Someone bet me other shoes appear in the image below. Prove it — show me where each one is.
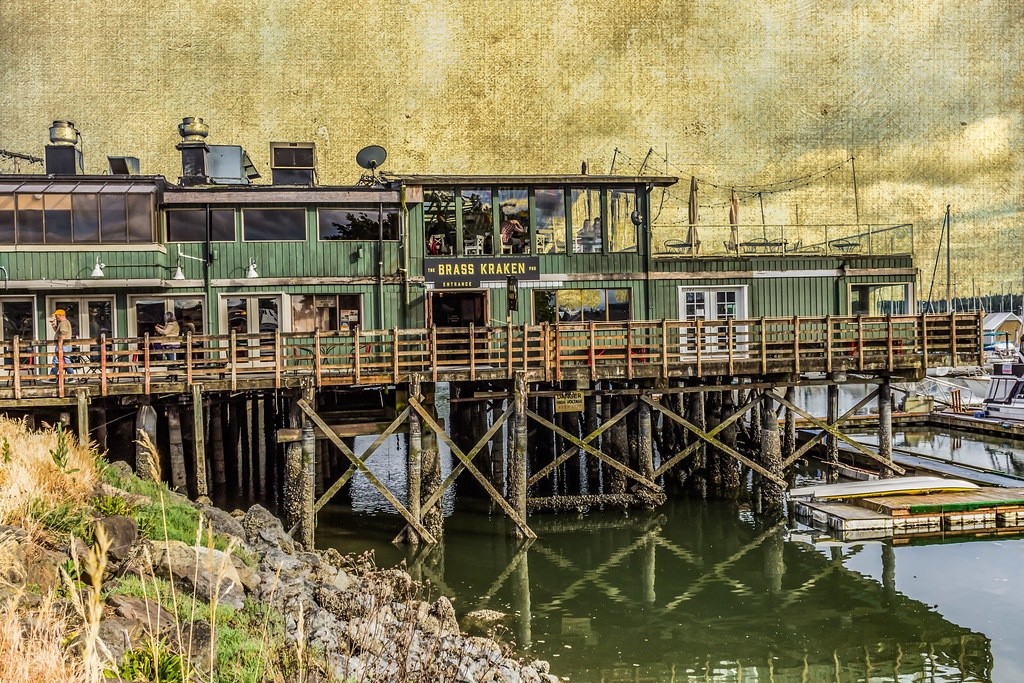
[65,380,75,384]
[43,380,57,384]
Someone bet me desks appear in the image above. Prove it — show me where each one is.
[738,243,788,253]
[667,244,697,254]
[833,243,860,254]
[313,344,342,375]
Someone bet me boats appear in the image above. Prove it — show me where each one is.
[855,331,1024,479]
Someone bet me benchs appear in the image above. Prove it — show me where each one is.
[849,341,904,356]
[587,349,647,367]
[169,352,228,382]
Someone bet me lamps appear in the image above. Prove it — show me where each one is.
[92,255,106,277]
[172,258,186,279]
[245,257,260,278]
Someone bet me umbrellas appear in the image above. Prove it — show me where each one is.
[686,177,698,254]
[730,190,739,251]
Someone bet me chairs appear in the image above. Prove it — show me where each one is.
[292,342,376,374]
[664,236,864,254]
[425,233,603,253]
[6,350,141,382]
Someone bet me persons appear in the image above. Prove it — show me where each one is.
[579,217,601,240]
[183,316,197,359]
[155,311,180,382]
[41,309,76,384]
[501,215,524,253]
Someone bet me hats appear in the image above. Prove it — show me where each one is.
[52,309,66,316]
